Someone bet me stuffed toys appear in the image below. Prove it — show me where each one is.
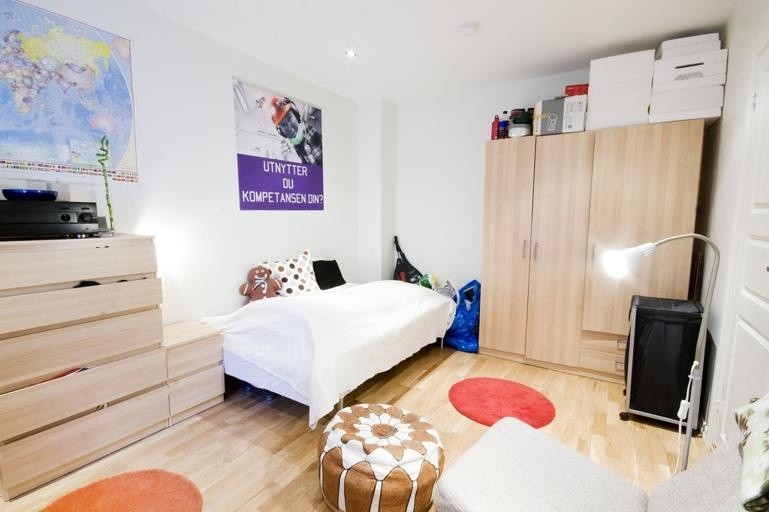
[238,265,284,306]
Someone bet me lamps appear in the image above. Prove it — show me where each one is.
[597,233,722,472]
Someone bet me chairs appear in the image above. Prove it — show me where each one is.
[427,393,769,511]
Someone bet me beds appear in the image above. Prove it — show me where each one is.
[201,278,458,429]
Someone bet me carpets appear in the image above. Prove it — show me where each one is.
[449,377,556,429]
[40,468,204,512]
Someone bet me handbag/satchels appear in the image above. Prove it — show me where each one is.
[443,280,480,353]
[431,279,461,307]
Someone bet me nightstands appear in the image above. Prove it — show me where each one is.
[161,318,226,428]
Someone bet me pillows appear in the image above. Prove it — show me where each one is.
[734,393,769,512]
[312,259,346,290]
[259,250,319,297]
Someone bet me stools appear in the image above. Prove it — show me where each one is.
[317,403,445,512]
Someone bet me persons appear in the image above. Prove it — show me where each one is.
[268,95,323,167]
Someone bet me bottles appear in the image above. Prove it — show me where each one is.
[491,109,509,140]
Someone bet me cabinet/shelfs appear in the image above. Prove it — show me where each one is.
[0,229,167,502]
[477,118,702,385]
[618,295,704,436]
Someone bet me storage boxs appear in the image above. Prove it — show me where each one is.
[532,32,729,136]
